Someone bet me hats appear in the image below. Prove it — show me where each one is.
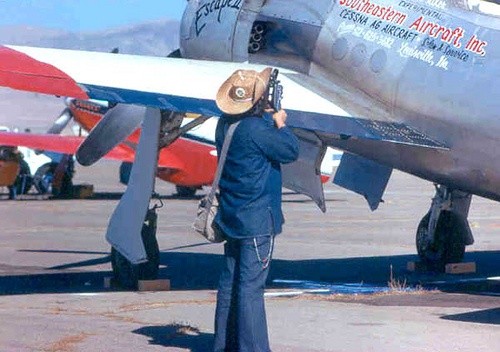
[215,67,273,116]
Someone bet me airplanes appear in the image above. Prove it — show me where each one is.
[0,0,500,287]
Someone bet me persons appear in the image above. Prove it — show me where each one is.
[0,145,34,200]
[209,67,301,352]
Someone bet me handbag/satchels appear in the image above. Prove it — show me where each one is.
[192,190,225,243]
